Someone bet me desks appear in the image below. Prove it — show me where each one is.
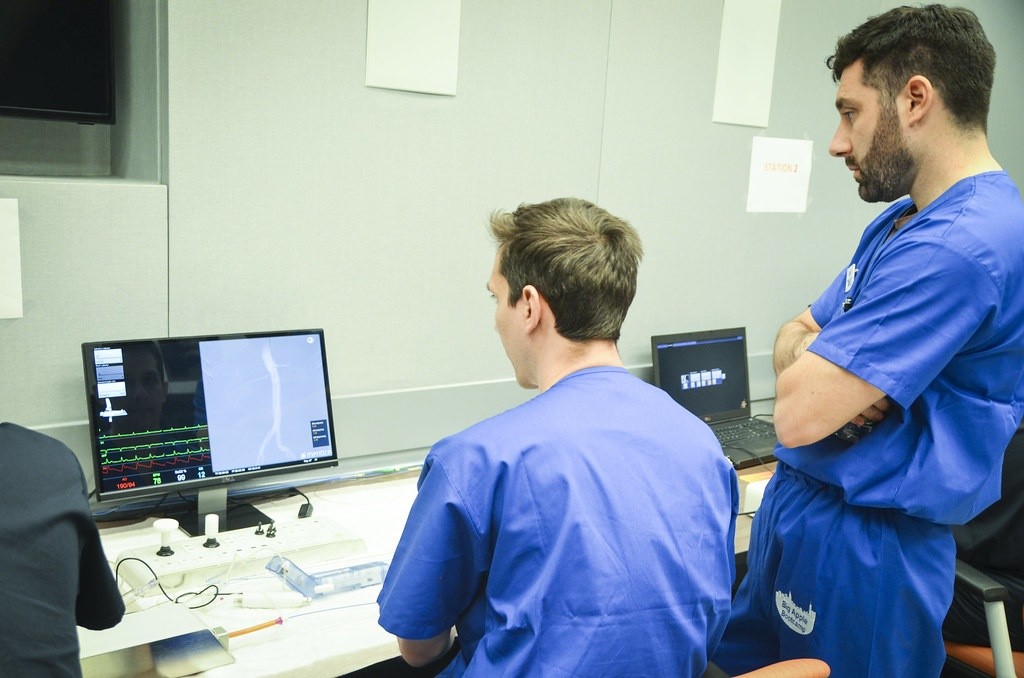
[76,455,779,678]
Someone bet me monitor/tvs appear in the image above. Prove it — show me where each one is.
[82,329,339,539]
[0,0,117,125]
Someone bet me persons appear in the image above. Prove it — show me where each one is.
[720,4,1024,678]
[0,421,126,678]
[376,199,739,678]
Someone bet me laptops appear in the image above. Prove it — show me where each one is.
[652,327,784,470]
